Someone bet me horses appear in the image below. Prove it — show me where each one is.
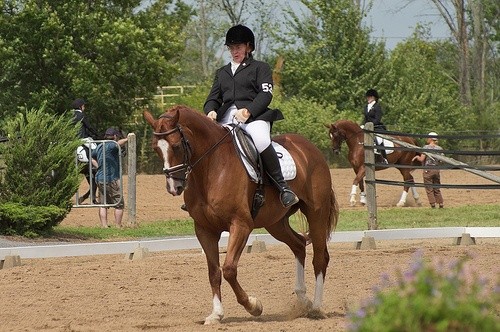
[76,125,126,206]
[139,103,340,325]
[322,117,425,208]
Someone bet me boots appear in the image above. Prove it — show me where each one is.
[259,143,299,209]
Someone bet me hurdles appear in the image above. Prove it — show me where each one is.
[364,121,500,230]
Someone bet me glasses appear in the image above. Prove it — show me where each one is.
[226,45,243,52]
[113,134,119,139]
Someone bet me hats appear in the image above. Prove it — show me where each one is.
[428,132,438,141]
[104,125,123,135]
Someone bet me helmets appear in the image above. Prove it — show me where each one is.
[72,97,87,109]
[365,88,379,103]
[224,24,256,53]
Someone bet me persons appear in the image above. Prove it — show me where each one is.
[417,132,443,208]
[202,24,298,207]
[90,127,128,228]
[360,89,389,165]
[72,98,99,150]
